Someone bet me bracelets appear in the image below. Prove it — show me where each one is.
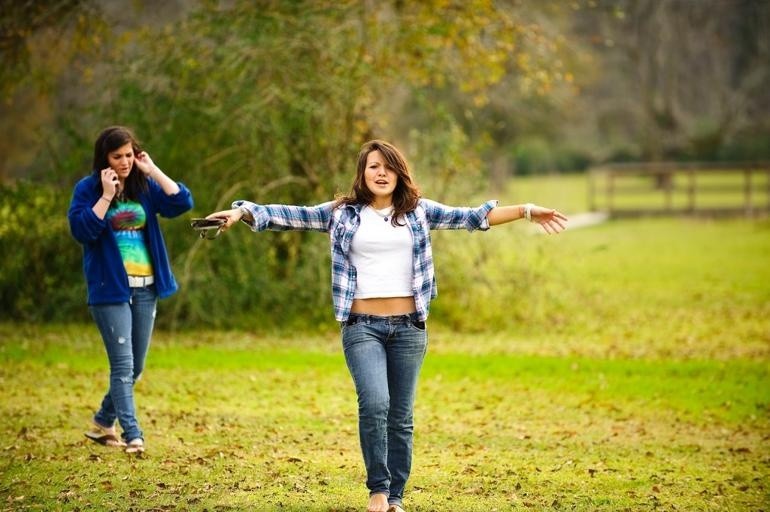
[239,207,248,218]
[526,202,534,221]
[101,196,111,203]
[519,204,526,218]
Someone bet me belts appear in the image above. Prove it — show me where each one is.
[127,275,155,288]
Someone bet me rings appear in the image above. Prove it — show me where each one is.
[111,174,116,178]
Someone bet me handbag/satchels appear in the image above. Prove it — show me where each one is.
[190,217,228,231]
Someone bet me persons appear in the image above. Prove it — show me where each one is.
[68,126,194,452]
[205,140,570,512]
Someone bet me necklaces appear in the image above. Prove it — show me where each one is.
[370,205,394,221]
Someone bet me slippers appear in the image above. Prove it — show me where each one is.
[84,431,126,446]
[126,444,144,454]
